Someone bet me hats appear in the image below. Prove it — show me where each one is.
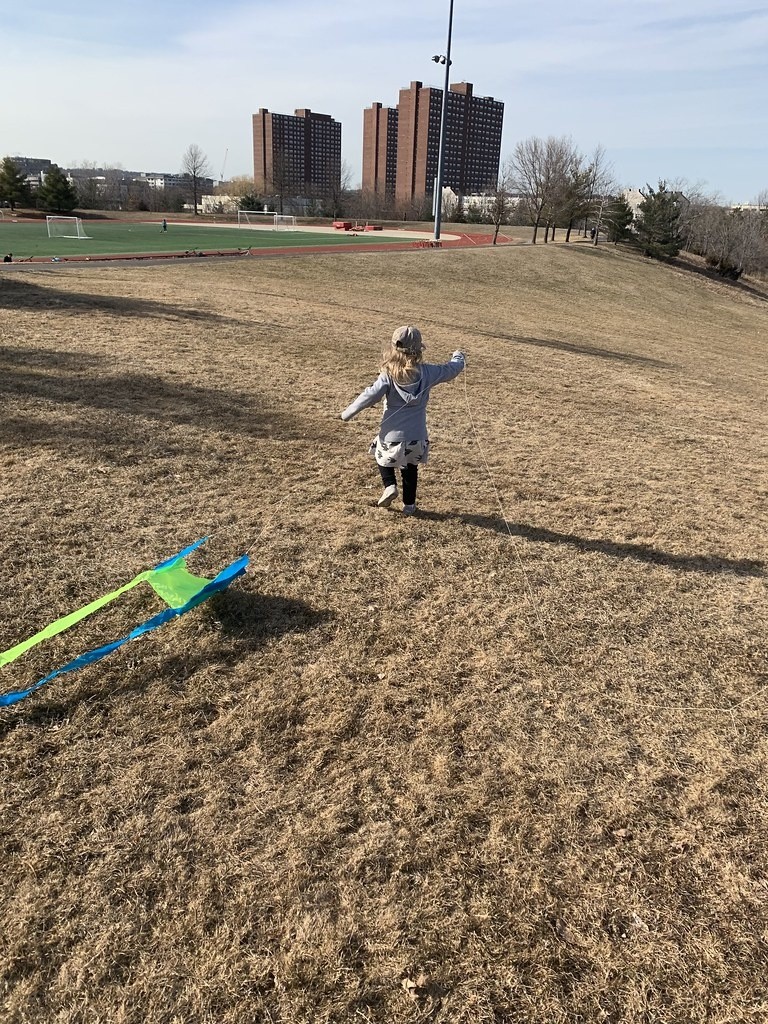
[391,326,422,352]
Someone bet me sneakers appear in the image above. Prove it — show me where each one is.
[403,504,416,515]
[378,485,399,507]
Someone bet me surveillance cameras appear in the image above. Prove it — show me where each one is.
[440,56,445,64]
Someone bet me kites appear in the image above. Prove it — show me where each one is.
[0,533,250,707]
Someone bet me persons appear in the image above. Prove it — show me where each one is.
[162,219,167,233]
[4,253,13,262]
[340,324,466,515]
[589,226,596,244]
